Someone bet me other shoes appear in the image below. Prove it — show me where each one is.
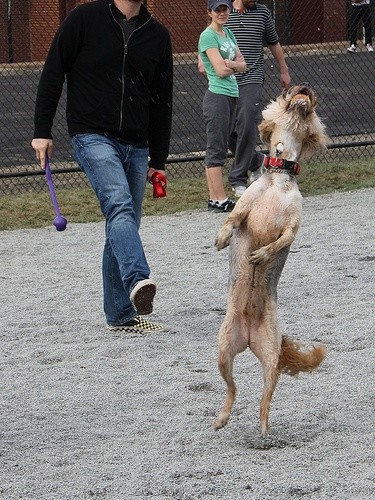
[129,279,156,315]
[366,43,374,52]
[347,44,356,53]
[109,315,163,332]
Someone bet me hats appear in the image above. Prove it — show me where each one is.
[208,0,231,11]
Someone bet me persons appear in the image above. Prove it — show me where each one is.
[198,0,291,197]
[347,0,374,53]
[198,0,246,213]
[31,0,174,332]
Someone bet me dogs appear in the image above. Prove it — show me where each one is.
[210,83,327,439]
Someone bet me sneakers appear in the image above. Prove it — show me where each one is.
[232,185,247,198]
[250,154,266,181]
[208,198,236,213]
